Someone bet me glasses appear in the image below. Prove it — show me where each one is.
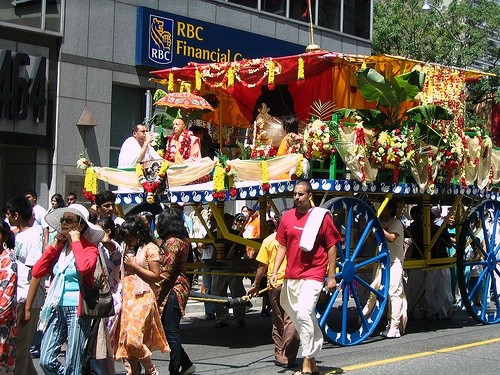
[138,129,149,133]
[60,217,75,224]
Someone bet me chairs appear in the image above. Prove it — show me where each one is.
[310,111,359,180]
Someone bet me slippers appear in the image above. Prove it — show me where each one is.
[291,369,319,375]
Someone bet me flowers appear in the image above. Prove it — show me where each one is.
[76,149,98,202]
[136,58,500,203]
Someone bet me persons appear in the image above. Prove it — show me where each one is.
[191,201,278,325]
[355,203,500,337]
[245,179,343,375]
[0,190,195,375]
[118,118,215,190]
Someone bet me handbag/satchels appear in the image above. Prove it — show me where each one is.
[81,255,115,318]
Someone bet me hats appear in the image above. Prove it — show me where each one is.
[45,204,105,243]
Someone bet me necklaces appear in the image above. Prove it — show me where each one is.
[184,135,191,159]
[163,129,189,160]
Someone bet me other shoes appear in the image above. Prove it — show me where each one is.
[180,364,197,375]
[199,315,212,320]
[29,346,39,359]
[215,322,228,328]
[284,364,298,369]
[238,321,246,327]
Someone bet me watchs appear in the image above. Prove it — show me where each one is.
[252,282,259,287]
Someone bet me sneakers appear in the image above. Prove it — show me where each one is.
[380,328,400,337]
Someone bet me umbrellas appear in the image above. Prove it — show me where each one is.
[153,92,215,111]
